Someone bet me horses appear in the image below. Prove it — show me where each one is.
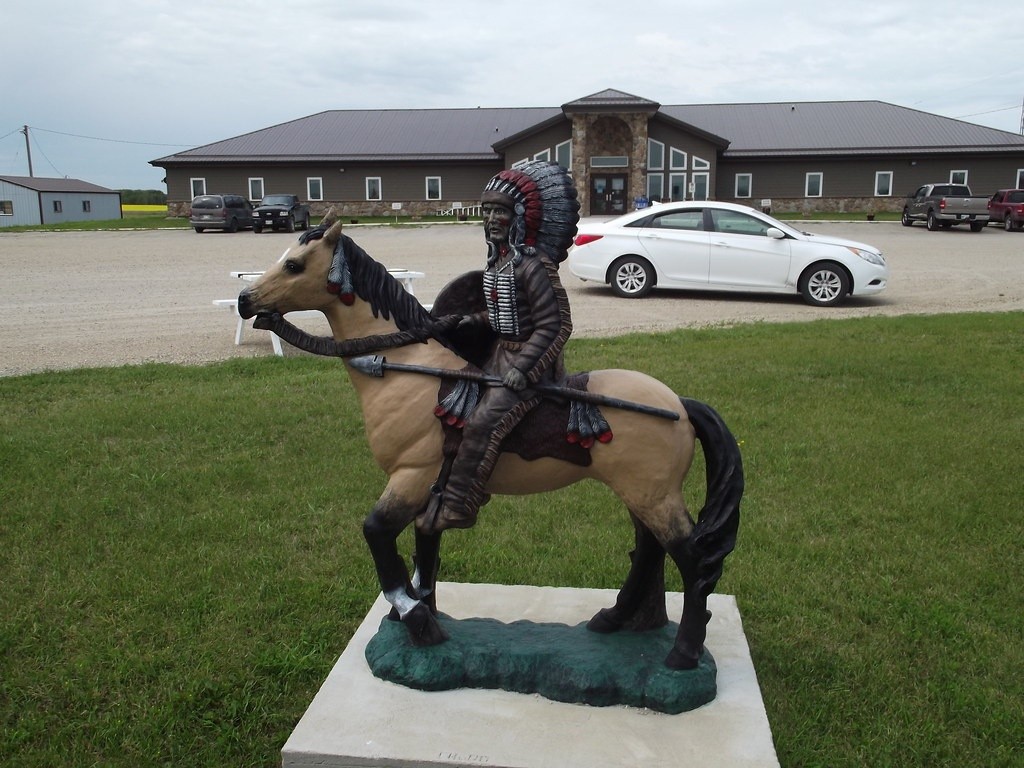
[234,204,747,671]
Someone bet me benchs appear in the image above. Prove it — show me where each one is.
[211,299,432,318]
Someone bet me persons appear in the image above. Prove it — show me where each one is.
[414,170,574,536]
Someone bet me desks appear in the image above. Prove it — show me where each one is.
[231,269,426,356]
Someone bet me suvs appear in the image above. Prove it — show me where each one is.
[189,196,257,233]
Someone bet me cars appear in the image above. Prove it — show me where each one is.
[566,200,889,306]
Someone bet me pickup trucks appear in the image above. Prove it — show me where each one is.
[251,194,312,232]
[902,182,992,233]
[990,189,1024,232]
